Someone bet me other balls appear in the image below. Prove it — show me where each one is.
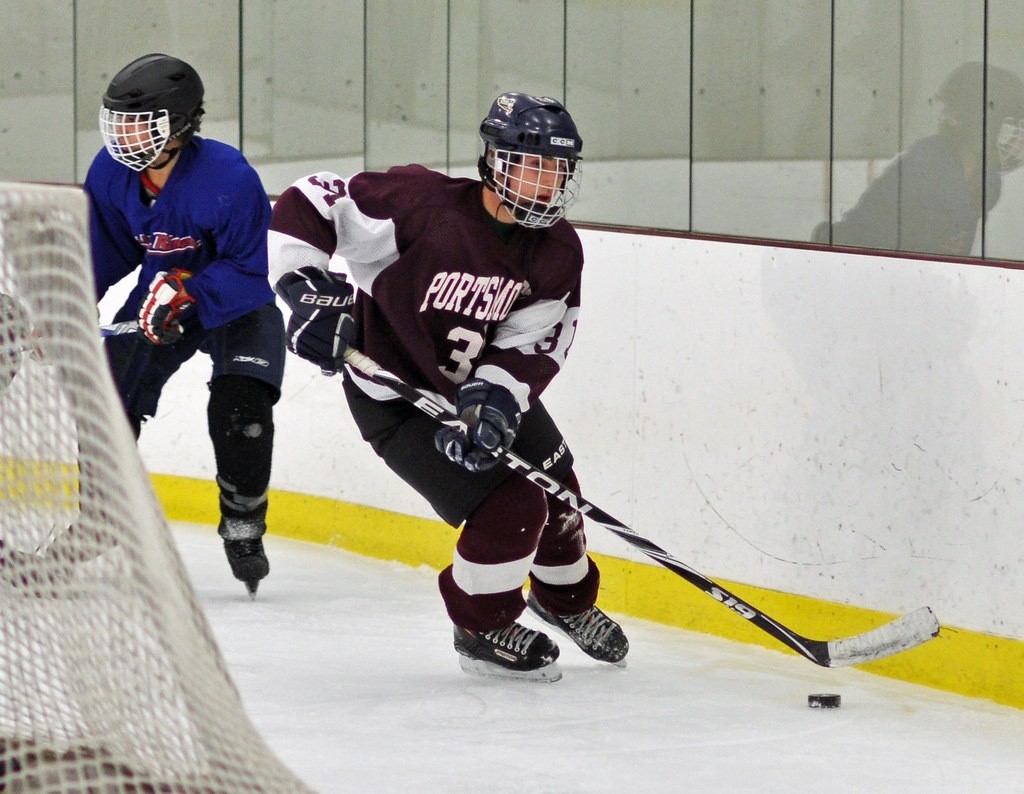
[808,693,842,709]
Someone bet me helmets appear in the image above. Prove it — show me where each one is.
[98,54,204,172]
[479,94,585,230]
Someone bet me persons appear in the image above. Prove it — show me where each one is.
[268,92,629,683]
[809,61,1024,255]
[21,54,286,600]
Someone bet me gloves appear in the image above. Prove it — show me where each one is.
[273,268,356,375]
[136,269,193,349]
[433,379,521,472]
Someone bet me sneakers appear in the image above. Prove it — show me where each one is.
[526,594,630,667]
[217,513,269,599]
[453,619,562,683]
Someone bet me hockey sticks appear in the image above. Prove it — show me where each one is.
[344,345,940,667]
